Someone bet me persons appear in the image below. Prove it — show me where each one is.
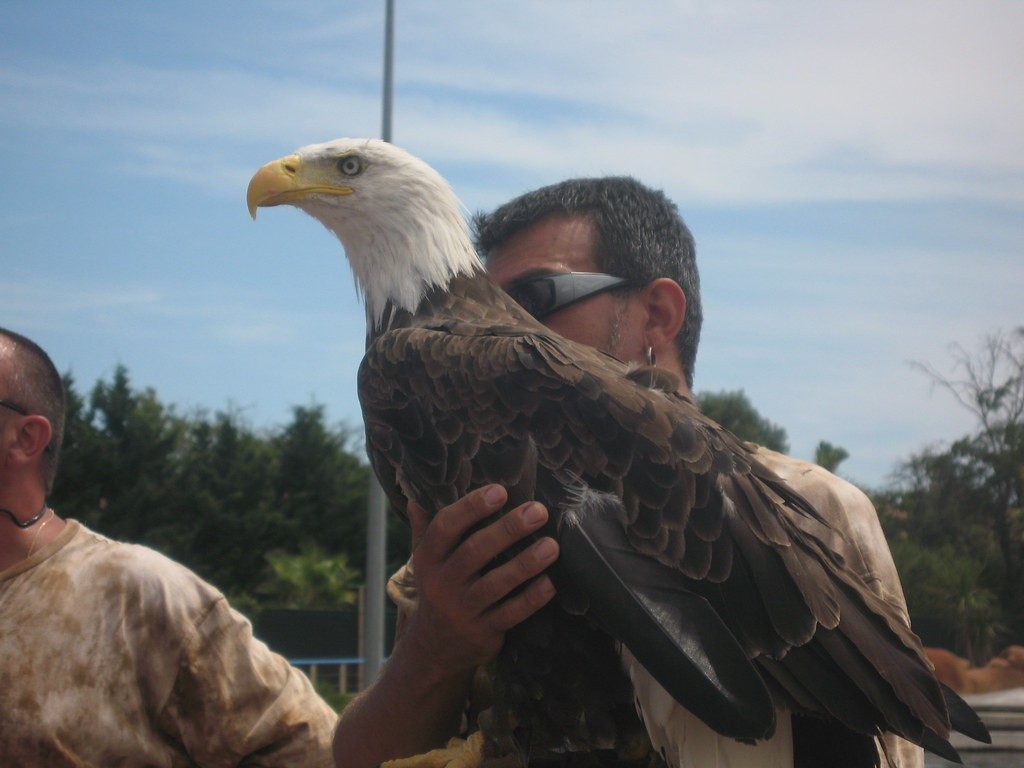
[330,177,925,768]
[0,323,340,768]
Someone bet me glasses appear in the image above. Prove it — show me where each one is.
[509,272,634,317]
